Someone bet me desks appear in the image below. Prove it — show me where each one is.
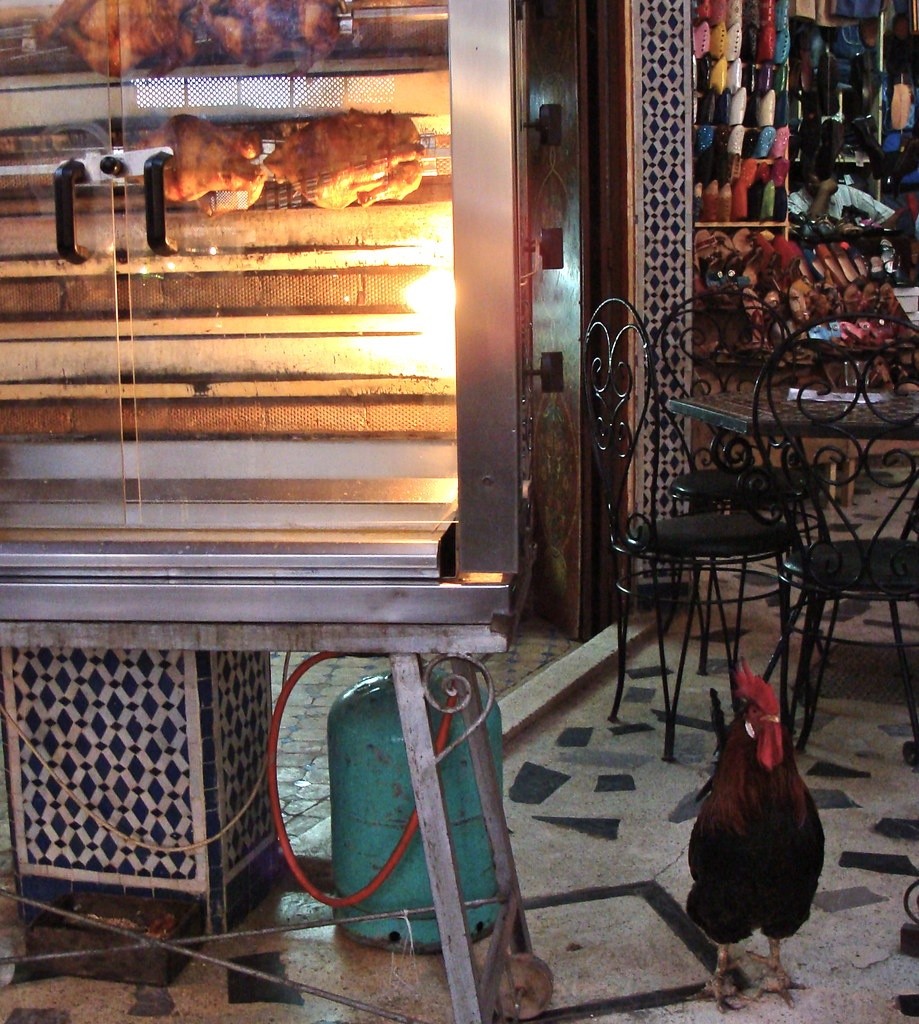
[665,370,918,753]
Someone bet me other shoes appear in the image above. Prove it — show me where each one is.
[693,0,916,366]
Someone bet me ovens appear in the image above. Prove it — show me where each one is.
[1,0,529,659]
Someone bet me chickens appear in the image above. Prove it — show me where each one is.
[43,0,339,81]
[158,106,425,217]
[685,657,825,1015]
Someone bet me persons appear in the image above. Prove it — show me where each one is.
[788,146,899,227]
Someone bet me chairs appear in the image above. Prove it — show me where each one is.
[578,276,919,770]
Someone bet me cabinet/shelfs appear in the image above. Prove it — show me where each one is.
[691,0,883,310]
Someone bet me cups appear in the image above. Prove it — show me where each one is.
[846,360,871,391]
